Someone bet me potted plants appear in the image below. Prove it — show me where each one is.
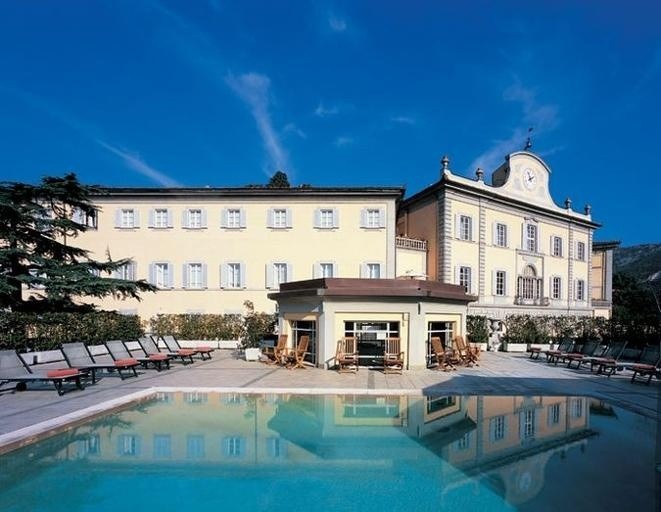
[237,300,278,360]
[467,314,618,355]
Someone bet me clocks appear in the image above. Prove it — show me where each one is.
[523,167,538,189]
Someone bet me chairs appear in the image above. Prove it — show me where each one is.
[431,335,483,371]
[384,337,406,375]
[335,337,360,374]
[530,337,661,386]
[0,333,216,396]
[259,334,311,369]
